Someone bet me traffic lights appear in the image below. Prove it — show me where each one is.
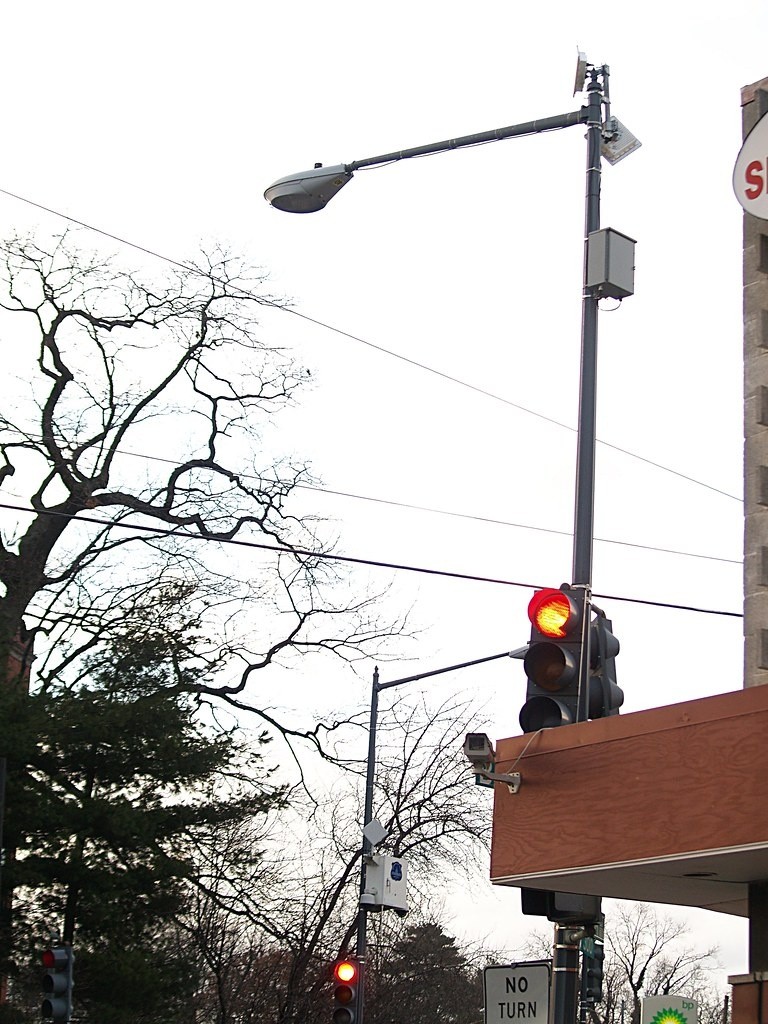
[41,940,74,1024]
[329,961,356,1024]
[588,606,624,720]
[517,586,587,734]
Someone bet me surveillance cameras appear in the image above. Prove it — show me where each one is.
[464,732,496,766]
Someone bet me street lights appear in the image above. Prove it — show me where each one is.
[354,645,528,1024]
[263,44,644,1024]
